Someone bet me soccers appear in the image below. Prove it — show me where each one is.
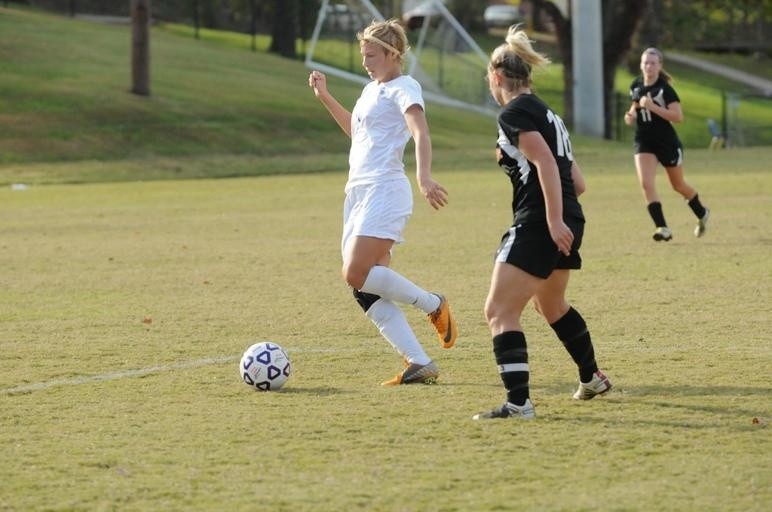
[239,341,291,391]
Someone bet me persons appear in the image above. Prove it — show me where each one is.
[470,21,614,424]
[305,18,468,390]
[622,47,713,246]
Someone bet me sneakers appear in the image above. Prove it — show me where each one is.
[653,227,672,242]
[695,207,709,238]
[427,290,457,349]
[380,360,440,386]
[472,397,535,420]
[573,369,612,400]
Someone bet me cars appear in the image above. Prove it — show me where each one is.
[400,0,446,30]
[322,3,374,32]
[482,4,518,29]
